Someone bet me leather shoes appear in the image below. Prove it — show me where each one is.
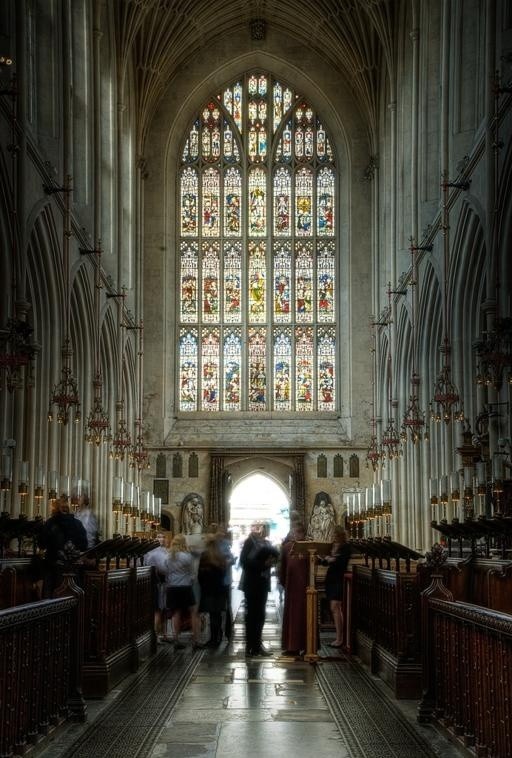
[326,642,342,648]
[162,635,273,656]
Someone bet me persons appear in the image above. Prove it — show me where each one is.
[73,493,98,550]
[132,521,280,657]
[304,500,336,543]
[317,524,352,649]
[37,497,88,559]
[180,497,204,535]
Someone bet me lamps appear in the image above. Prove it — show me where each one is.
[380,409,402,462]
[5,313,38,395]
[469,316,510,397]
[83,370,111,445]
[424,329,465,428]
[129,416,153,472]
[395,371,430,447]
[46,337,83,429]
[108,400,131,463]
[362,416,386,474]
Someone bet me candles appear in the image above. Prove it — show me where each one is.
[347,480,392,516]
[113,477,162,518]
